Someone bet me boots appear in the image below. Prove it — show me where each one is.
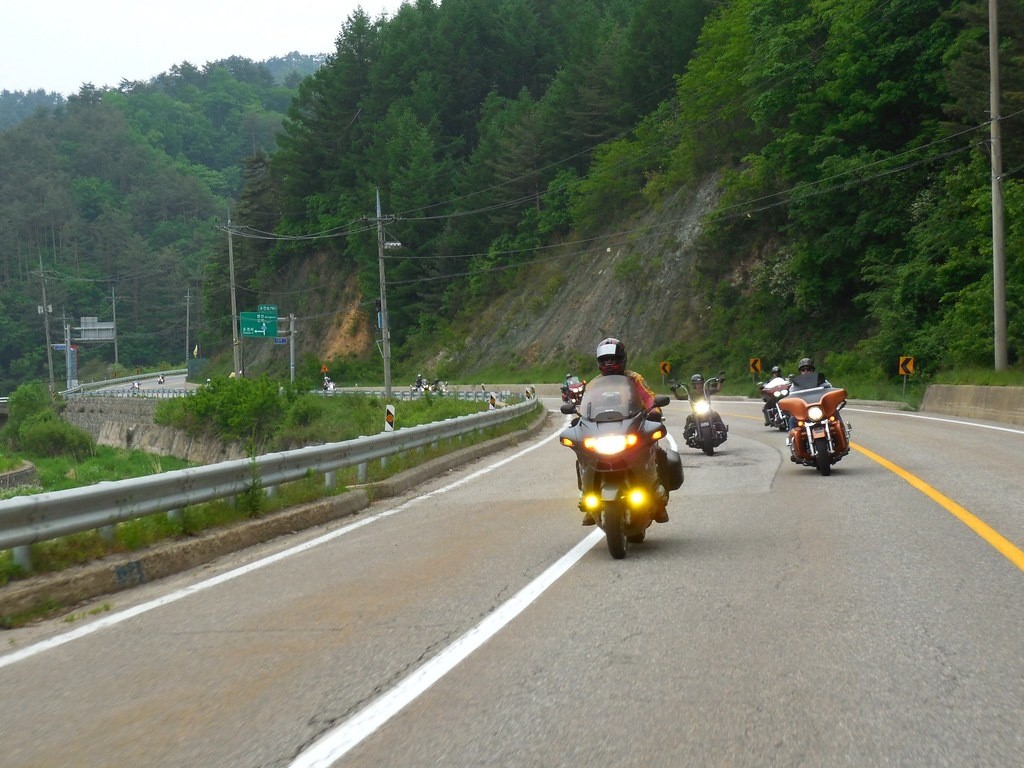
[765,417,770,426]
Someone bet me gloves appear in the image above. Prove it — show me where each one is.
[719,378,724,383]
[647,411,661,422]
[670,386,676,392]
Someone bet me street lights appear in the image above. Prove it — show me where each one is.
[378,240,403,401]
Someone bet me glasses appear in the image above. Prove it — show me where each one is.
[801,367,812,372]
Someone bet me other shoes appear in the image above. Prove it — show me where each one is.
[582,513,596,526]
[686,439,691,445]
[791,455,796,462]
[656,508,669,523]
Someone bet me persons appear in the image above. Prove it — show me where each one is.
[572,338,670,526]
[670,373,727,445]
[560,373,582,403]
[131,374,212,388]
[324,374,446,393]
[760,358,849,462]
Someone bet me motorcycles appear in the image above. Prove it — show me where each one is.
[410,379,429,392]
[558,374,684,563]
[775,371,852,476]
[757,374,795,432]
[323,381,336,391]
[429,381,448,392]
[666,378,728,457]
[131,382,141,394]
[157,375,164,384]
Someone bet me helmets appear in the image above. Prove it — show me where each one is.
[771,366,781,374]
[597,338,627,376]
[325,376,329,379]
[798,358,815,371]
[566,373,573,378]
[691,374,704,383]
[582,380,587,385]
[418,374,422,377]
